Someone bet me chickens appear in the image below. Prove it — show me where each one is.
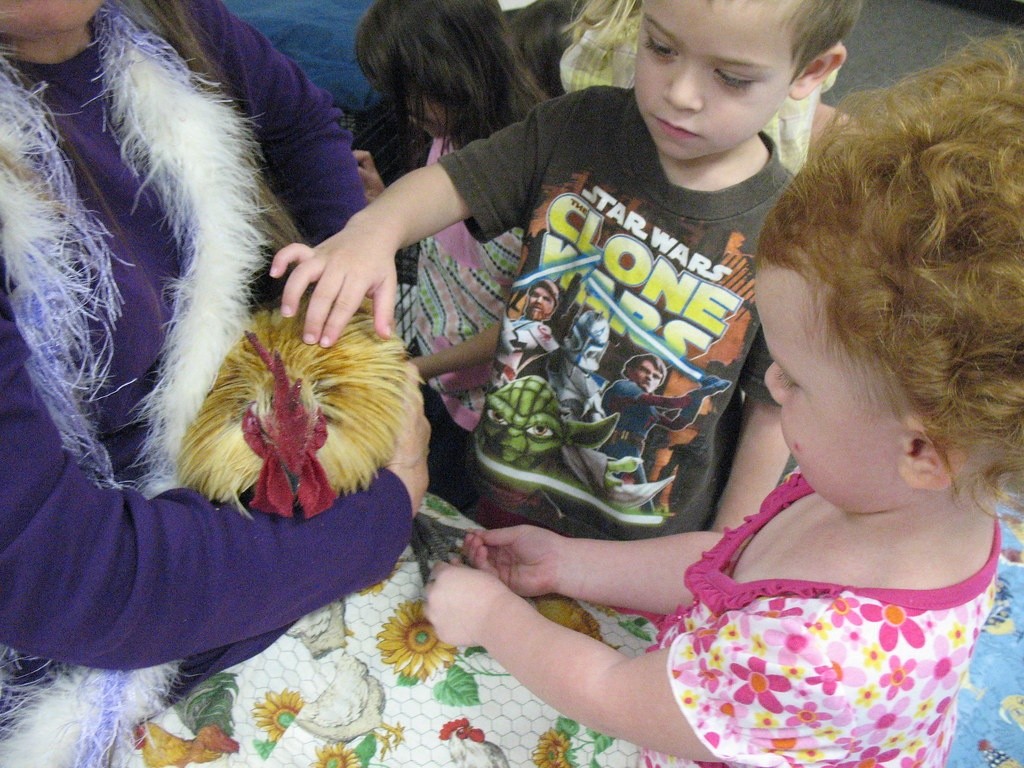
[177,288,424,521]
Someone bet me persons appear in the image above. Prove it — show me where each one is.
[0,0,431,768]
[16,303,692,768]
[274,0,869,535]
[426,39,1024,768]
[349,0,854,456]
